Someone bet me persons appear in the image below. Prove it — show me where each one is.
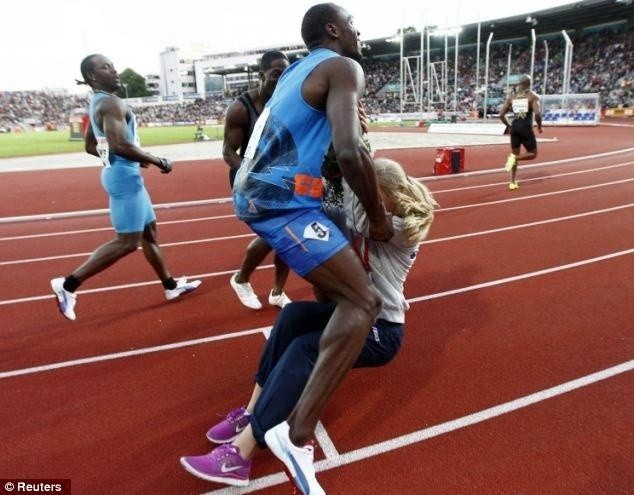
[1,25,634,144]
[221,49,293,311]
[179,97,442,488]
[50,52,204,322]
[231,2,396,495]
[499,74,545,190]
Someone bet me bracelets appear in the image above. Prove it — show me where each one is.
[160,157,169,170]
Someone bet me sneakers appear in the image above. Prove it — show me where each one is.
[509,182,518,190]
[504,153,517,172]
[164,274,202,300]
[179,442,252,486]
[50,277,77,320]
[268,289,292,309]
[230,272,262,309]
[206,404,252,443]
[264,420,326,494]
